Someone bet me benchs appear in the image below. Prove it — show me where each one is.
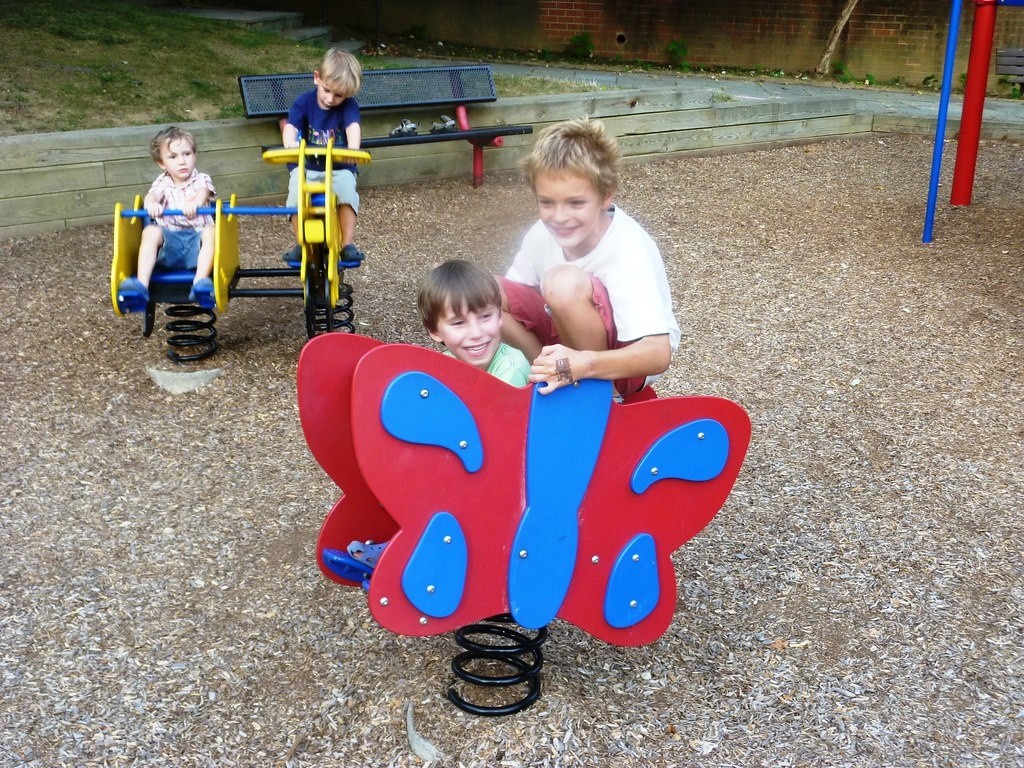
[238,65,532,189]
[996,47,1024,93]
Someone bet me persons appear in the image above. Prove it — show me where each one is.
[281,47,366,263]
[118,126,217,303]
[346,259,534,570]
[491,118,681,403]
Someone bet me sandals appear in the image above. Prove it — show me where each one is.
[119,277,149,303]
[282,245,302,261]
[187,277,213,301]
[339,244,365,262]
[429,115,456,134]
[388,119,417,138]
[346,540,390,568]
